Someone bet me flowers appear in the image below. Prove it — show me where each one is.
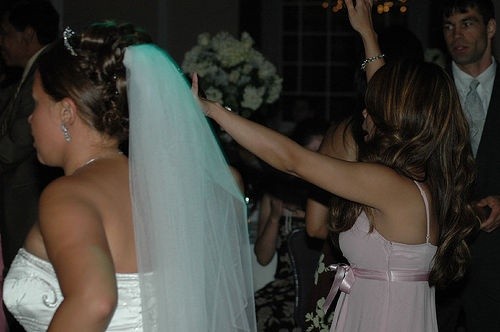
[181,31,283,143]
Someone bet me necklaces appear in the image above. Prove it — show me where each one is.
[73,151,123,173]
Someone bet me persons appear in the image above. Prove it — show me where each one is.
[229,116,325,331]
[3,20,257,332]
[434,0,500,332]
[0,0,66,332]
[305,25,426,240]
[191,0,461,332]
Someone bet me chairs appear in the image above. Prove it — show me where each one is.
[287,227,350,332]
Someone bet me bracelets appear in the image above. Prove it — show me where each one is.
[360,54,386,70]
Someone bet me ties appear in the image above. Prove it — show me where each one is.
[465,80,484,159]
